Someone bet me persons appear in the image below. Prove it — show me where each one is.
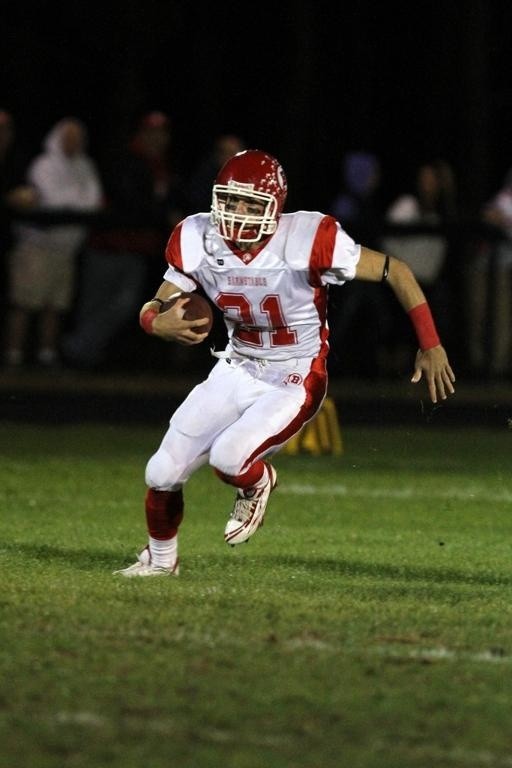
[112,147,460,576]
[0,103,512,370]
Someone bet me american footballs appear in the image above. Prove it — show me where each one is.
[159,291,213,334]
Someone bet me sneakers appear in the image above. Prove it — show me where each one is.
[111,544,181,577]
[224,459,278,545]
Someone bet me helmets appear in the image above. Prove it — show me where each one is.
[209,148,287,244]
